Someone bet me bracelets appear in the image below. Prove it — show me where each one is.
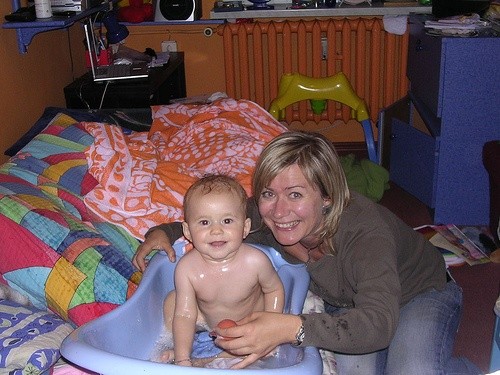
[173,359,193,365]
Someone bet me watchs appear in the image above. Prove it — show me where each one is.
[293,315,305,346]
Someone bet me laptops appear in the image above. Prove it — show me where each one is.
[84,23,148,82]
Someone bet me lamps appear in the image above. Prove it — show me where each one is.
[101,9,129,49]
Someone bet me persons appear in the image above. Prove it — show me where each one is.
[132,131,484,375]
[156,174,284,367]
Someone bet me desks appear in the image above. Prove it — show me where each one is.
[64,52,187,111]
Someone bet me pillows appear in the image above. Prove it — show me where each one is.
[4,106,102,156]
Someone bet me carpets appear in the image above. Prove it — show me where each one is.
[446,260,500,372]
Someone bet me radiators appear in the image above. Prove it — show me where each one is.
[215,17,408,127]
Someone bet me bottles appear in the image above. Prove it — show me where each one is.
[34,0,53,18]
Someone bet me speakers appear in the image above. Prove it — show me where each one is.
[154,0,197,23]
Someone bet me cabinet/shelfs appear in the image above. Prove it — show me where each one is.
[377,14,500,226]
